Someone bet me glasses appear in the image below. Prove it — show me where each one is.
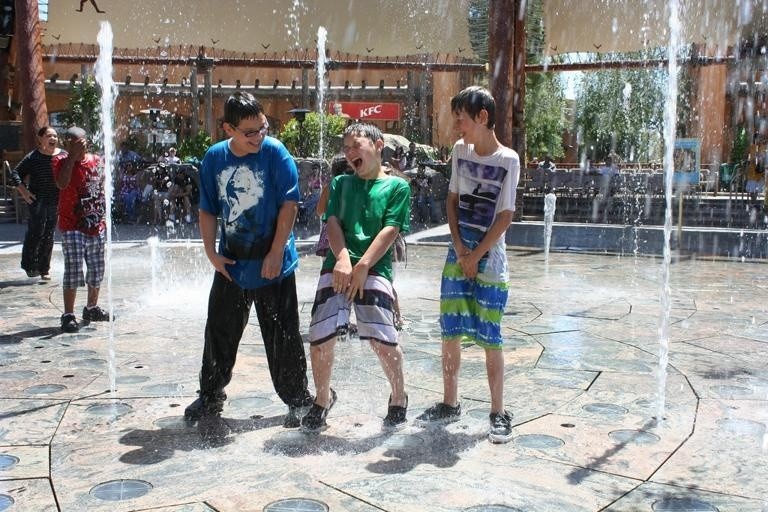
[176,172,183,175]
[228,117,270,138]
[126,164,131,167]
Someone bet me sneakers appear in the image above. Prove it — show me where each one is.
[186,214,193,224]
[381,392,408,430]
[185,392,225,419]
[61,313,80,333]
[300,388,336,432]
[42,273,51,281]
[284,396,315,427]
[490,408,513,442]
[26,269,39,277]
[414,402,461,427]
[83,305,115,321]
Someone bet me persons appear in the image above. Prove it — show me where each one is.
[536,154,556,195]
[302,143,455,236]
[413,84,520,443]
[51,126,115,332]
[299,123,410,431]
[116,137,198,229]
[184,90,312,421]
[10,126,70,281]
[313,150,410,338]
[594,155,622,199]
[745,130,766,204]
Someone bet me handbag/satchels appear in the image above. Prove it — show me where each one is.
[192,179,199,205]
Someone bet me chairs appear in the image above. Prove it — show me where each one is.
[526,163,735,199]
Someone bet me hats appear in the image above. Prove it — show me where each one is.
[178,166,187,174]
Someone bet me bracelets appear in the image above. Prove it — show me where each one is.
[21,189,29,196]
